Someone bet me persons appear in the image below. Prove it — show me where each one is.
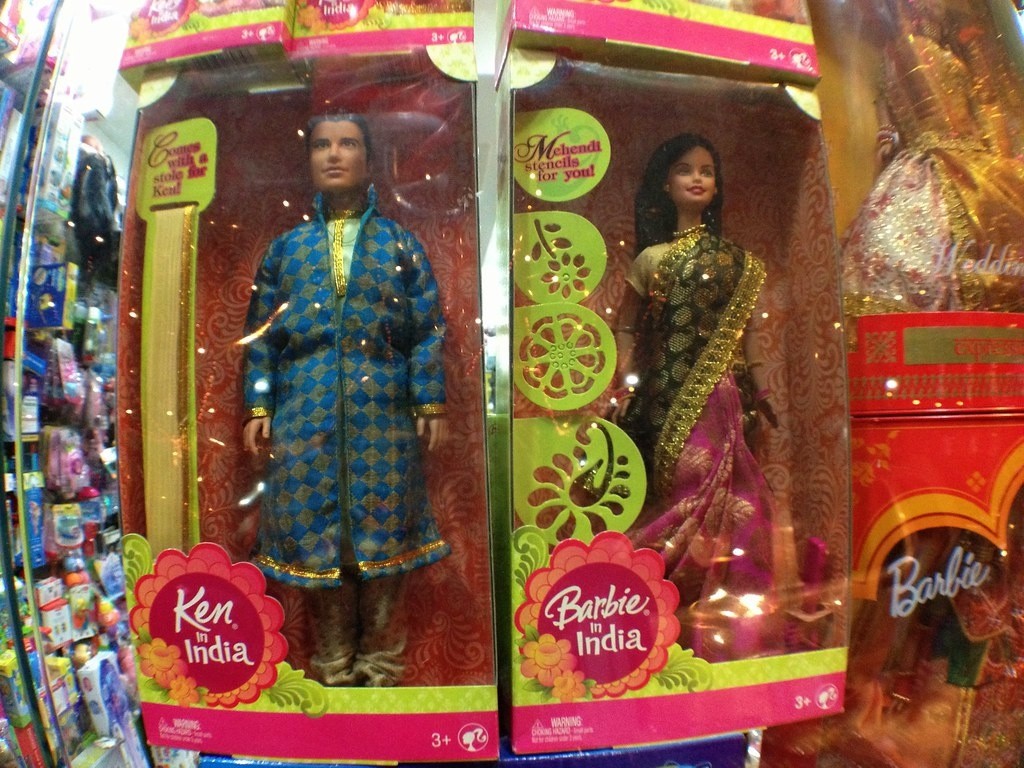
[239,111,450,687]
[607,131,778,667]
[840,0,1024,317]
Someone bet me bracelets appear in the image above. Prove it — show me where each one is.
[616,325,635,333]
[746,358,765,369]
[614,385,635,401]
[757,389,774,401]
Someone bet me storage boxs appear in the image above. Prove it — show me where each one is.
[119,0,1024,767]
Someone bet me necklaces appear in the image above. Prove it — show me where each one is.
[876,123,898,146]
[670,223,707,237]
